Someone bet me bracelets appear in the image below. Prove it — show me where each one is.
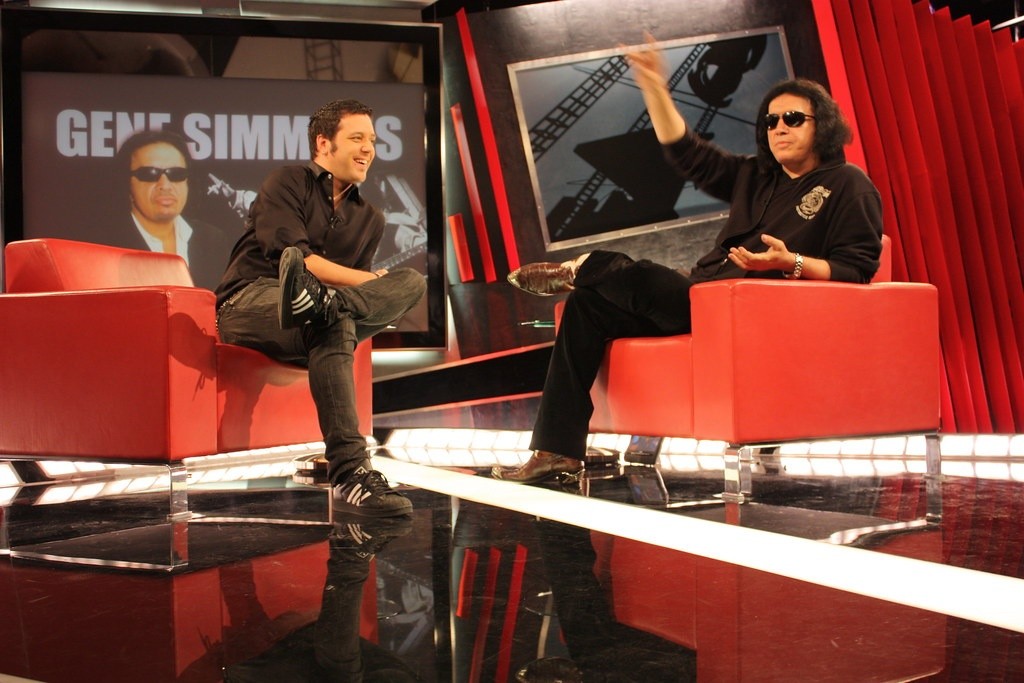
[375,273,382,277]
[782,253,803,279]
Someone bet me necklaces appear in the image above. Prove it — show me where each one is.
[333,183,353,201]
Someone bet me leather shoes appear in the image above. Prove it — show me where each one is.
[491,449,585,484]
[507,255,581,297]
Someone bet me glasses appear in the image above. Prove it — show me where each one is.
[129,166,190,182]
[759,111,816,130]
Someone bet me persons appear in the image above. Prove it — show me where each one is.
[213,100,426,517]
[491,28,883,485]
[310,518,415,683]
[95,128,229,290]
[518,516,697,683]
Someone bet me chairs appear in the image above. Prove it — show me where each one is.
[586,530,950,683]
[0,239,377,523]
[554,233,945,551]
[0,539,379,683]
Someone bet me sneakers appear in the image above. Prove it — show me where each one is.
[330,522,411,562]
[330,458,413,517]
[279,247,332,330]
